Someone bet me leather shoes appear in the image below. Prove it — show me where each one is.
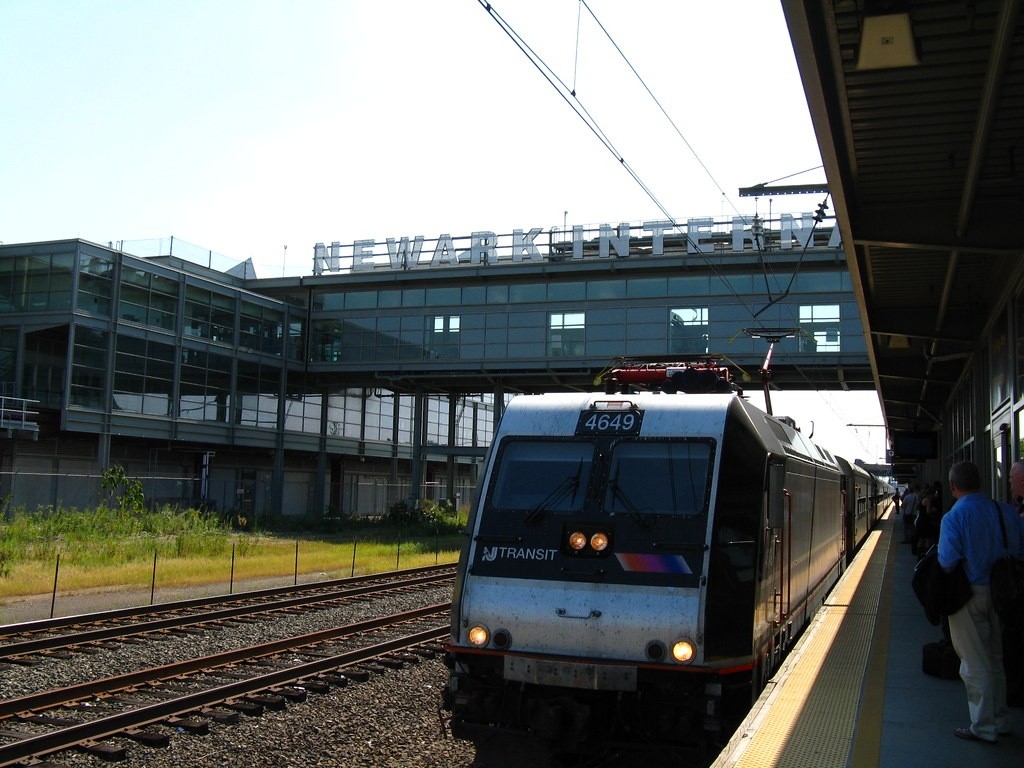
[952,727,999,745]
[996,731,1013,736]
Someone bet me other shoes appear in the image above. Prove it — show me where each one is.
[900,540,910,544]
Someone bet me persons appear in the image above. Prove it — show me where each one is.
[892,461,956,566]
[1002,461,1024,709]
[937,461,1024,745]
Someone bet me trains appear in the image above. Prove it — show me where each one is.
[440,351,898,763]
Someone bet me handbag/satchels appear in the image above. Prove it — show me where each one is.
[990,555,1024,621]
[902,513,913,525]
